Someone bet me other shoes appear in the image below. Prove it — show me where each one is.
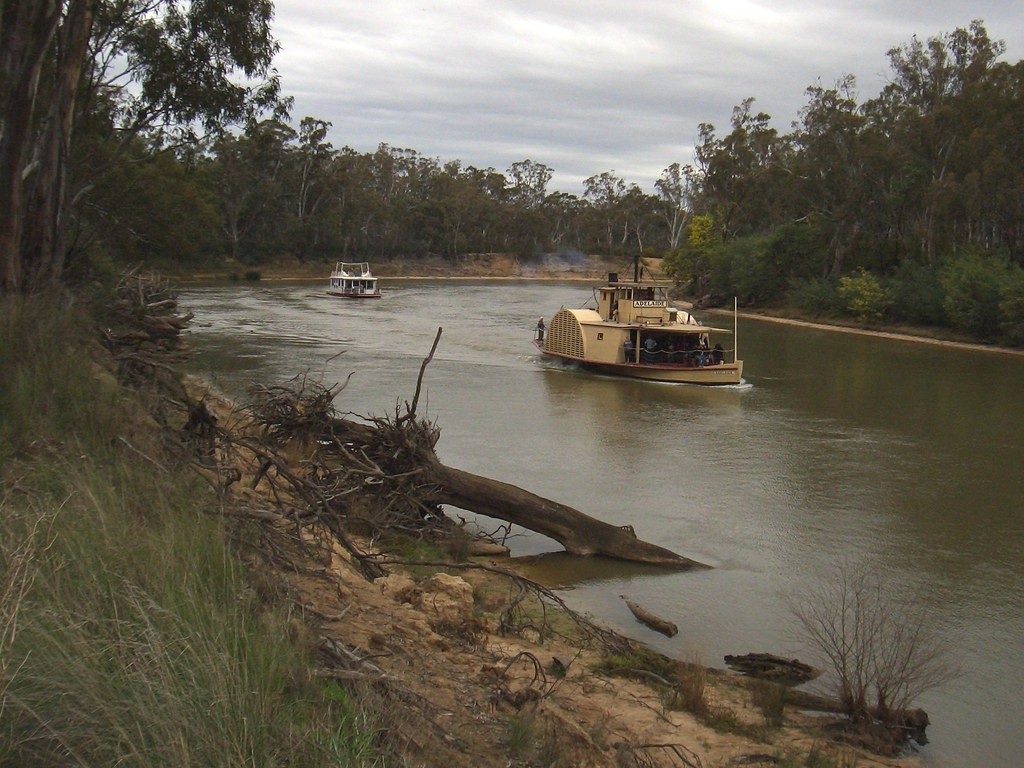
[624,361,633,364]
[537,338,544,341]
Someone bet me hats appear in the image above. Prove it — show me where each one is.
[540,316,543,319]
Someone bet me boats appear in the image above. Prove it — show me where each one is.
[325,261,381,299]
[532,257,745,388]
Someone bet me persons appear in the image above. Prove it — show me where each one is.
[645,337,657,365]
[624,336,633,364]
[538,317,546,339]
[685,342,709,368]
[612,300,619,322]
